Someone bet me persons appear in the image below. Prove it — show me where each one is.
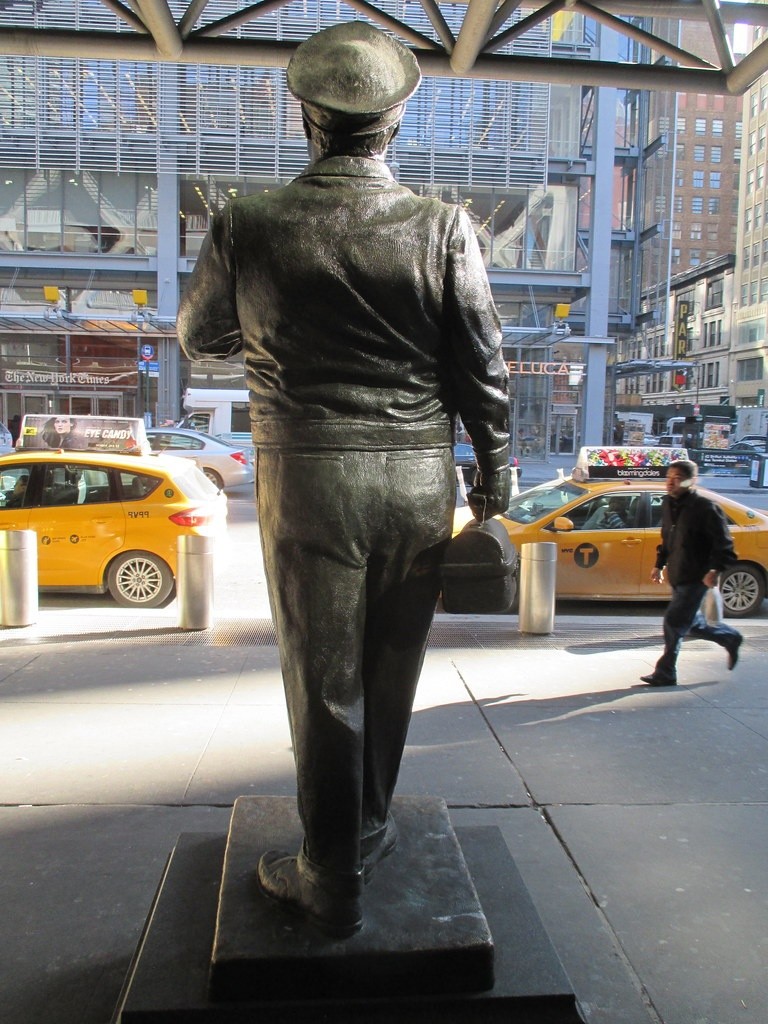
[42,416,81,449]
[6,475,29,508]
[175,21,514,938]
[518,429,534,457]
[640,459,743,687]
[618,424,624,444]
[560,432,566,449]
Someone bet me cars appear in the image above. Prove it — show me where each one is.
[0,414,230,608]
[444,446,768,616]
[454,443,523,488]
[86,428,256,491]
[0,422,12,455]
[643,434,659,446]
[725,435,767,468]
[551,434,573,451]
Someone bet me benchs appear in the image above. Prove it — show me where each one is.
[131,477,145,497]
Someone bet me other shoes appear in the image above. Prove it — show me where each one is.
[727,634,742,668]
[640,673,676,686]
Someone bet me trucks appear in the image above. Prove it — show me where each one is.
[166,388,254,464]
[617,412,654,435]
[729,407,768,445]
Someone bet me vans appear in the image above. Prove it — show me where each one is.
[654,435,684,449]
[667,418,703,439]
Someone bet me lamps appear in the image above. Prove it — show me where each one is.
[43,286,59,305]
[555,303,570,321]
[132,290,148,308]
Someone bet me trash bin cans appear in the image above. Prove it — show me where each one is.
[749,452,768,488]
